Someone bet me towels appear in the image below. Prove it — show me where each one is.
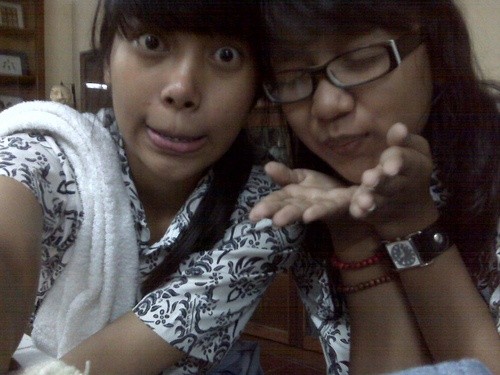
[1,99,142,359]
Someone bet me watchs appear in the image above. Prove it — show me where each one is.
[381,210,455,270]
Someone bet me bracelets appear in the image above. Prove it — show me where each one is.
[329,252,388,273]
[334,270,403,295]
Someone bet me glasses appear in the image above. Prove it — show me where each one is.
[259,29,424,106]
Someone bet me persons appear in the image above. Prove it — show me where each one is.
[247,0,500,373]
[2,1,305,373]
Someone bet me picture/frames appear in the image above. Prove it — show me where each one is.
[1,49,31,78]
[0,92,28,112]
[0,1,29,29]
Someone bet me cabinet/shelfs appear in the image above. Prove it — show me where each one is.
[240,270,325,350]
[0,2,47,113]
